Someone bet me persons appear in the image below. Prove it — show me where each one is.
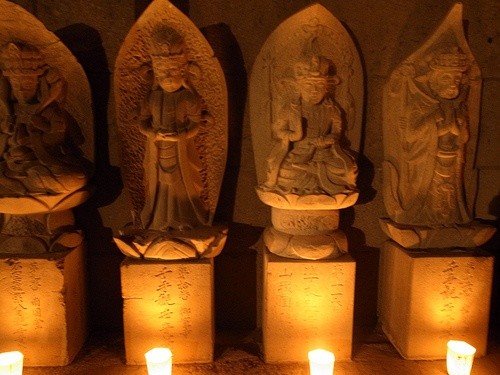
[399,45,473,227]
[130,31,213,231]
[0,40,87,197]
[265,50,359,193]
